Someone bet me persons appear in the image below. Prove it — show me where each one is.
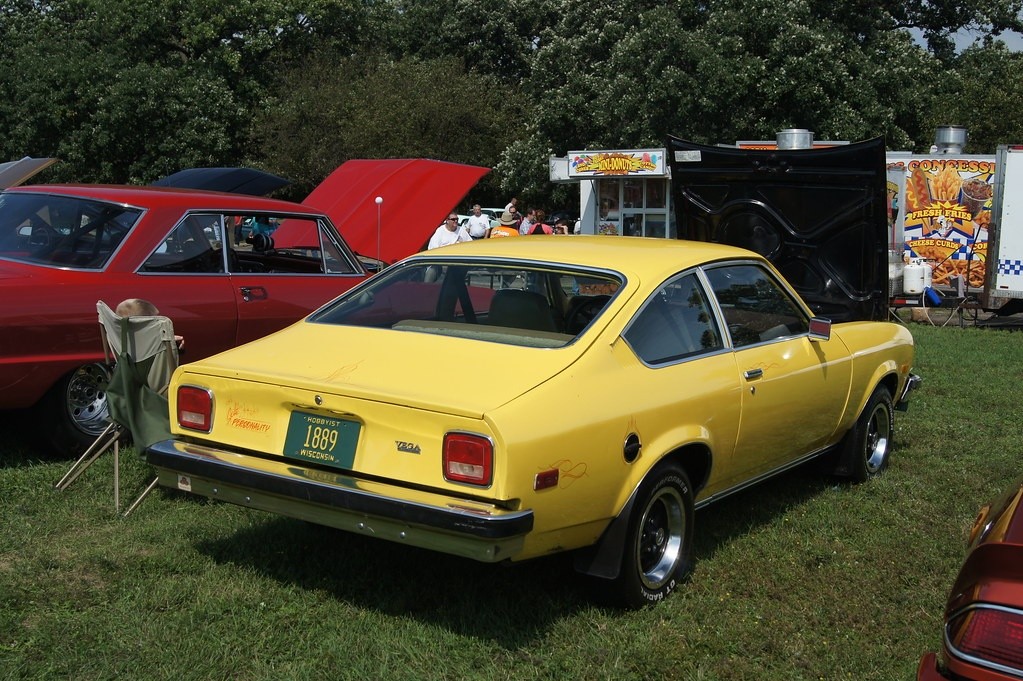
[504,196,584,235]
[489,212,520,239]
[464,204,491,241]
[248,215,271,252]
[116,298,187,357]
[234,215,246,248]
[427,210,472,254]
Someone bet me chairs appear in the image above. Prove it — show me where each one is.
[625,304,693,362]
[478,289,559,332]
[564,295,612,335]
[55,299,180,517]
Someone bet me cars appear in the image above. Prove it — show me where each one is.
[144,133,920,615]
[0,158,494,461]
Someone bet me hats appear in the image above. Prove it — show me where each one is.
[496,212,517,225]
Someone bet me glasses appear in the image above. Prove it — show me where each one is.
[448,218,459,221]
